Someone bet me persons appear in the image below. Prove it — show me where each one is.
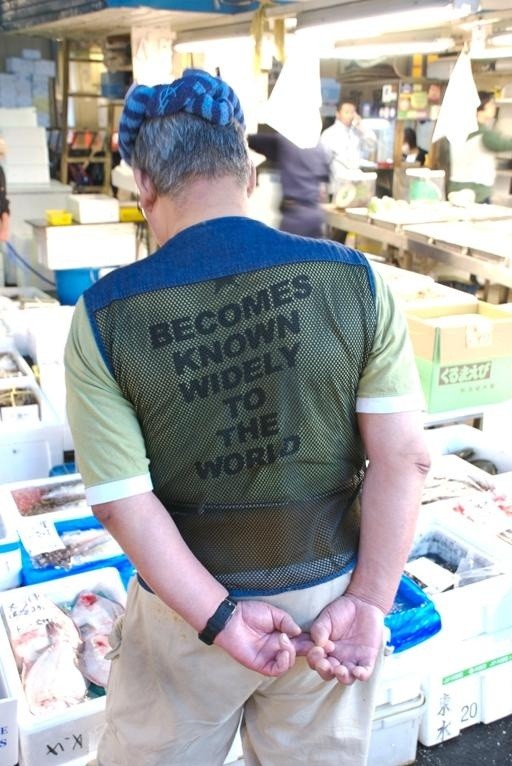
[0,138,9,242]
[321,100,378,172]
[243,106,333,240]
[402,126,427,163]
[63,69,433,766]
[448,91,512,205]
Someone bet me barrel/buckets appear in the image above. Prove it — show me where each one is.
[405,166,447,203]
[52,267,101,304]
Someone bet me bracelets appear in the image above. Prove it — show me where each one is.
[199,599,236,648]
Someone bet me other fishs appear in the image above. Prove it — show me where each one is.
[40,480,86,505]
[23,589,126,719]
